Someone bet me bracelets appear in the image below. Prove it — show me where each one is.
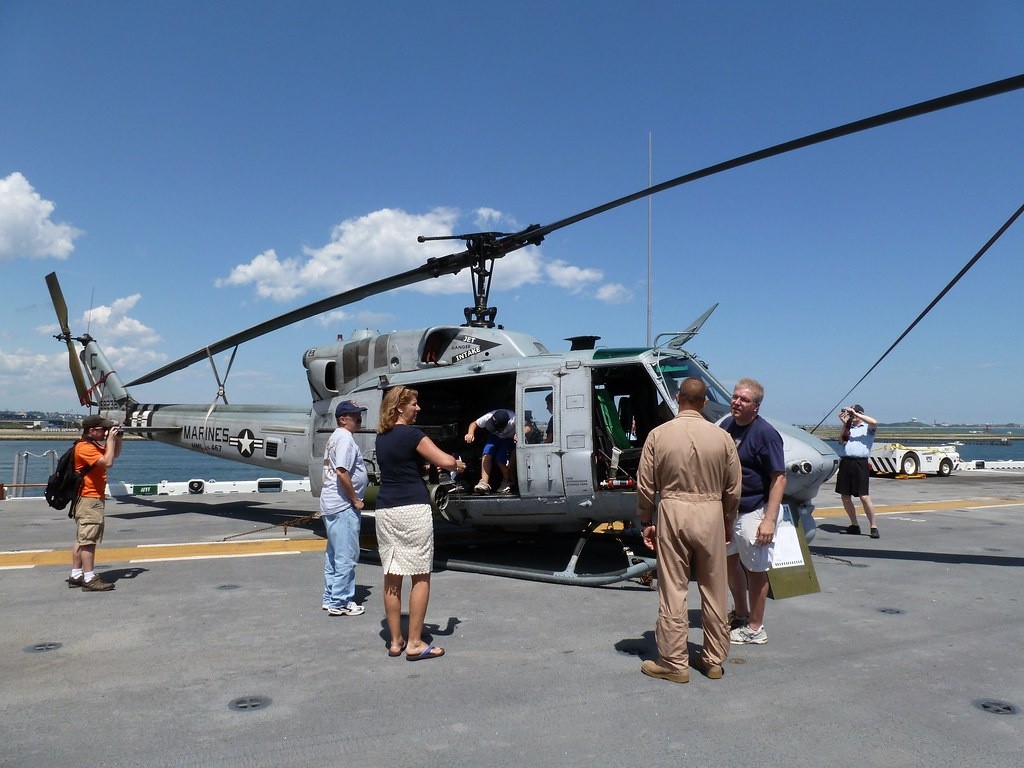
[640,521,653,527]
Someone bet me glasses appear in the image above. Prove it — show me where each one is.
[399,385,410,401]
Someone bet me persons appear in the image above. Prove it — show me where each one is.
[835,405,880,538]
[636,375,743,684]
[545,392,553,443]
[375,385,465,660]
[465,409,533,495]
[66,414,123,591]
[719,378,786,645]
[319,400,369,615]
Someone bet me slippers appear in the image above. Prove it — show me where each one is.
[389,640,407,656]
[406,644,445,661]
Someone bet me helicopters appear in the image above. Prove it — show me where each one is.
[43,75,1024,586]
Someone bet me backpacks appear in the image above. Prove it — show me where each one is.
[44,438,97,510]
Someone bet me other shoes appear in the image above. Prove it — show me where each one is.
[840,524,861,534]
[641,658,689,684]
[871,528,880,538]
[694,653,723,679]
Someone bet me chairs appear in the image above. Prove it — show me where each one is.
[595,390,642,479]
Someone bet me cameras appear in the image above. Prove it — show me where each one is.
[108,427,124,437]
[840,409,847,416]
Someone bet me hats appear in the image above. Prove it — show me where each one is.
[847,404,864,413]
[485,410,510,433]
[335,400,368,419]
[82,414,114,428]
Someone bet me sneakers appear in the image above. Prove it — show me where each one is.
[728,610,750,630]
[82,573,115,592]
[496,480,514,494]
[64,574,84,588]
[321,600,365,616]
[474,479,492,495]
[729,624,768,645]
